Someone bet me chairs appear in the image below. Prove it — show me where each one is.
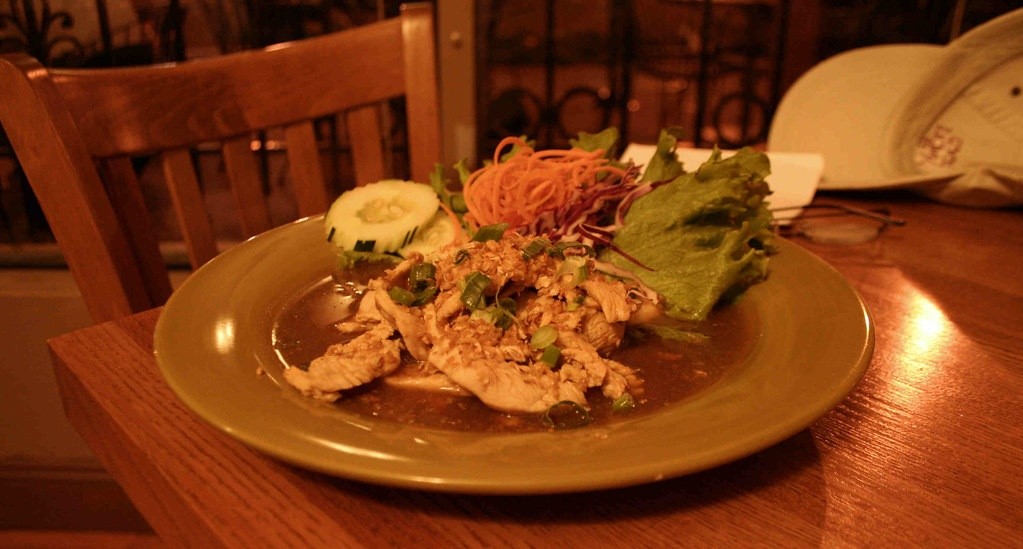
[0,0,443,327]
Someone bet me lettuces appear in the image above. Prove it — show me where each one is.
[430,123,778,321]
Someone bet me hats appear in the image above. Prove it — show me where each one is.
[766,9,1022,206]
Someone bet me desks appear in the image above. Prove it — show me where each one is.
[46,191,1023,549]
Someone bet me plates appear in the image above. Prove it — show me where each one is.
[152,210,876,495]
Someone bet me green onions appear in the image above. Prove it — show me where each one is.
[389,224,635,430]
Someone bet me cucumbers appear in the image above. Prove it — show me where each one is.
[323,178,454,265]
